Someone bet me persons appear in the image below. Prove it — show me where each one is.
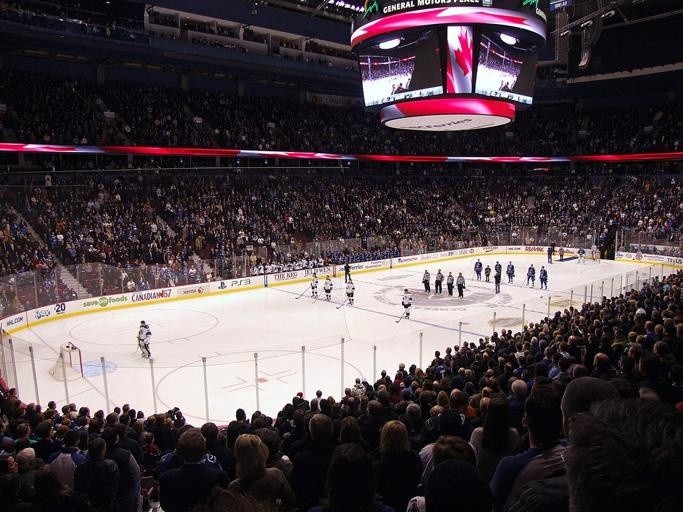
[595,249,601,264]
[558,245,564,261]
[422,270,430,294]
[506,261,514,284]
[2,153,300,317]
[499,80,516,91]
[539,266,547,289]
[547,245,556,264]
[1,378,304,511]
[310,273,319,298]
[526,264,535,288]
[474,259,482,281]
[495,261,501,272]
[522,271,681,337]
[577,247,585,265]
[494,271,501,293]
[137,321,152,359]
[434,268,445,295]
[361,61,412,81]
[479,50,520,77]
[2,12,359,72]
[391,83,405,94]
[301,329,681,511]
[344,263,352,283]
[456,272,465,299]
[446,271,454,296]
[323,274,333,301]
[591,242,597,261]
[442,96,680,157]
[3,68,441,157]
[502,164,681,256]
[300,161,503,270]
[485,265,491,282]
[402,288,412,319]
[346,279,355,305]
